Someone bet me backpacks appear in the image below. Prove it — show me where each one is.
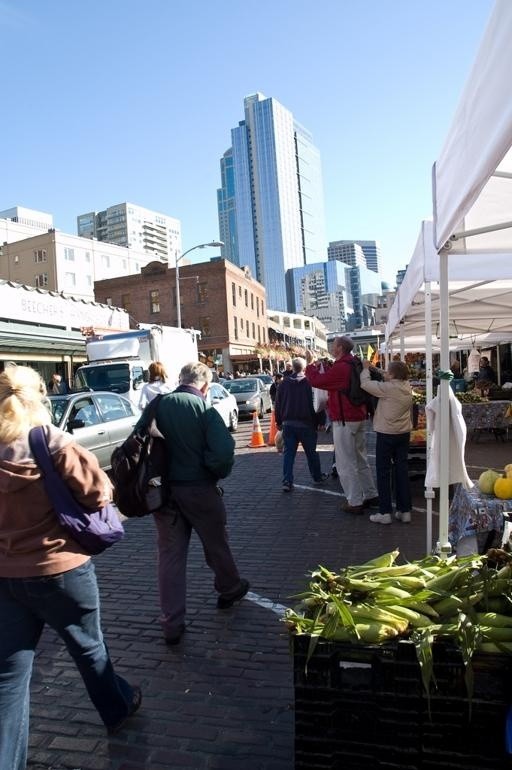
[111,394,167,517]
[334,356,384,426]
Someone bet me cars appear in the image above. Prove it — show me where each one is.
[48,391,139,471]
[221,378,270,418]
[246,375,273,389]
[218,378,226,384]
[210,383,239,431]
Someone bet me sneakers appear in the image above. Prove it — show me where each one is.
[281,479,293,492]
[312,473,329,485]
[339,501,411,524]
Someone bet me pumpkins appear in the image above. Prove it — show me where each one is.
[494,477,512,499]
[478,470,501,494]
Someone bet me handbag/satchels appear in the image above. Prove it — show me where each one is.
[29,426,124,554]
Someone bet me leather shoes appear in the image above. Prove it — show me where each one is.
[109,687,142,734]
[218,579,250,608]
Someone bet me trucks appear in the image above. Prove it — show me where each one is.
[73,327,201,406]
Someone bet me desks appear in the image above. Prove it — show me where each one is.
[449,479,512,547]
[462,400,512,444]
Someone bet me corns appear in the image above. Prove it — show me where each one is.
[311,550,512,654]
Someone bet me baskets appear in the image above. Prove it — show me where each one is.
[293,635,512,770]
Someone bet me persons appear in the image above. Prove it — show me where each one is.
[476,357,498,385]
[213,336,413,524]
[139,362,177,412]
[275,357,323,492]
[118,362,249,646]
[0,367,143,769]
[49,374,67,396]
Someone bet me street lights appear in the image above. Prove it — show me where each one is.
[175,240,225,326]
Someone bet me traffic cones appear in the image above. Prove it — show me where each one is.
[264,406,279,446]
[247,412,267,447]
